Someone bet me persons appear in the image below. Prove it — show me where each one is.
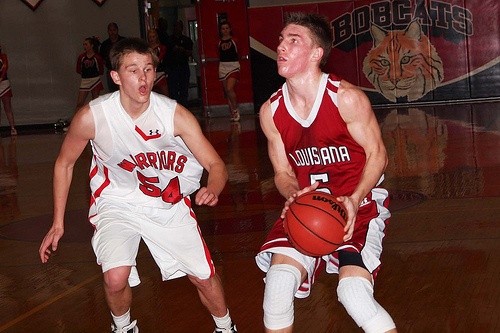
[216,24,241,124]
[255,12,398,333]
[99,22,131,94]
[141,29,170,97]
[63,36,104,133]
[167,21,193,105]
[38,37,238,333]
[0,47,17,135]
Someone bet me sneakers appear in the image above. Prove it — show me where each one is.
[11,129,17,134]
[229,109,241,121]
[110,321,140,333]
[213,321,238,333]
[231,122,241,134]
[10,137,16,141]
[63,127,69,131]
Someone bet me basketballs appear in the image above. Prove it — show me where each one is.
[283,191,350,258]
